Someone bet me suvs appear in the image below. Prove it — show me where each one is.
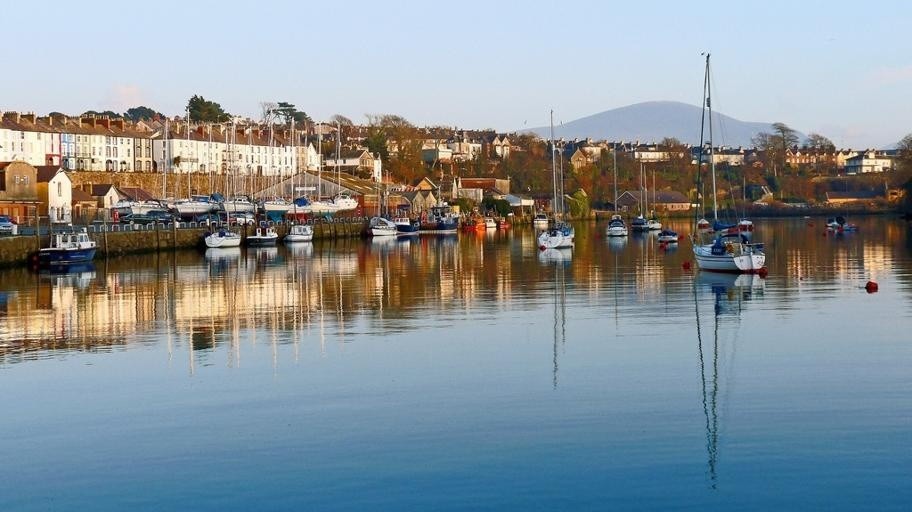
[0,215,15,234]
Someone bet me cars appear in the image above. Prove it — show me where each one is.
[226,213,255,225]
[197,214,218,226]
[119,211,154,225]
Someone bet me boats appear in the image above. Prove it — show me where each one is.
[246,227,278,247]
[204,228,241,248]
[37,233,97,265]
[283,224,314,241]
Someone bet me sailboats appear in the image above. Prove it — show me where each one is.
[112,109,359,219]
[534,108,575,248]
[824,181,858,230]
[605,143,678,243]
[689,52,766,270]
[366,151,511,235]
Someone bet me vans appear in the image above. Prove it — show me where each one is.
[146,211,181,225]
